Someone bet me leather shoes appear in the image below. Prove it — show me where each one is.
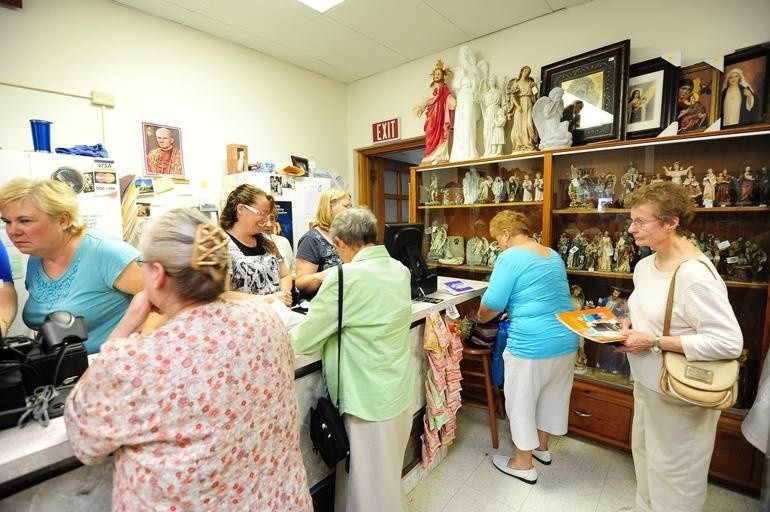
[532,449,552,465]
[492,454,537,484]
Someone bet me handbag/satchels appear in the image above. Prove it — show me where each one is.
[465,322,499,350]
[659,350,740,409]
[310,397,349,470]
[492,318,509,385]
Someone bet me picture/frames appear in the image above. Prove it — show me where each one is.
[142,122,187,180]
[538,38,770,146]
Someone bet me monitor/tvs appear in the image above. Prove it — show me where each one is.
[383,223,424,270]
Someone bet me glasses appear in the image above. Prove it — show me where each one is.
[133,256,148,266]
[244,204,270,219]
[624,219,661,230]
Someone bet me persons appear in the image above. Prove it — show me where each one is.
[272,177,280,193]
[410,160,770,375]
[146,126,183,175]
[261,203,293,275]
[63,202,315,512]
[621,180,744,512]
[472,208,580,484]
[288,201,417,512]
[296,160,308,175]
[0,240,19,341]
[295,187,353,294]
[231,147,248,173]
[0,177,153,354]
[223,182,293,307]
[411,45,573,165]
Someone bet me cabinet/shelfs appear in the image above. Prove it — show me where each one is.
[409,123,770,501]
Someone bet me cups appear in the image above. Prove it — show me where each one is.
[28,119,54,153]
